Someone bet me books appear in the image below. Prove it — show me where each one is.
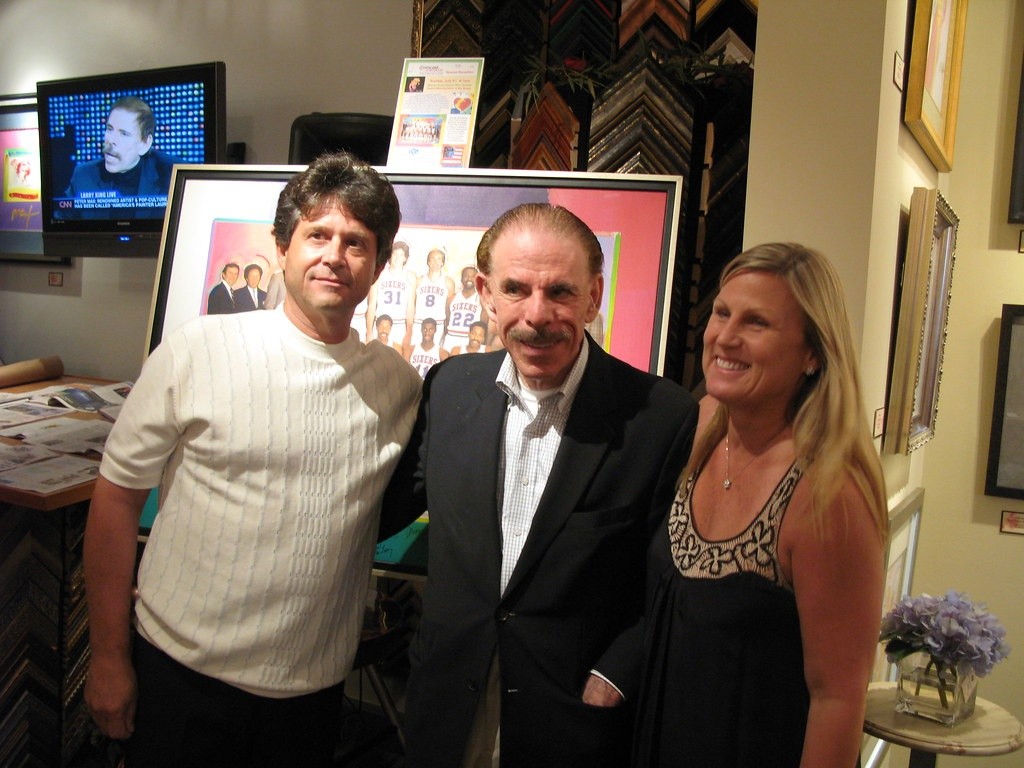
[0,380,134,495]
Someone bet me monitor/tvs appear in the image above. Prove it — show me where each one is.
[36,60,229,258]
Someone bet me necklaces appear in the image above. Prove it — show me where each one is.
[723,429,765,488]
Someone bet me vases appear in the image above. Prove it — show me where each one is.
[895,650,977,729]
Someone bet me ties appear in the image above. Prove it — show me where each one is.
[230,288,236,309]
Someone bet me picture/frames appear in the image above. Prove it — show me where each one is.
[140,165,684,583]
[984,303,1024,499]
[0,92,75,266]
[882,187,961,457]
[881,487,925,617]
[904,0,968,173]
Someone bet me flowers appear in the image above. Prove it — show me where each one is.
[878,588,1011,681]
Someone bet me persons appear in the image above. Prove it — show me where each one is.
[235,264,267,312]
[207,263,240,314]
[83,150,424,768]
[377,202,699,768]
[406,78,423,93]
[54,97,187,220]
[350,283,376,346]
[402,118,435,141]
[443,146,455,157]
[632,242,883,768]
[404,318,450,380]
[264,271,287,310]
[411,249,455,348]
[442,267,488,353]
[450,319,505,357]
[584,314,603,345]
[372,241,417,353]
[376,314,404,356]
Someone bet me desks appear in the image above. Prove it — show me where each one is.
[863,681,1024,768]
[0,373,134,768]
